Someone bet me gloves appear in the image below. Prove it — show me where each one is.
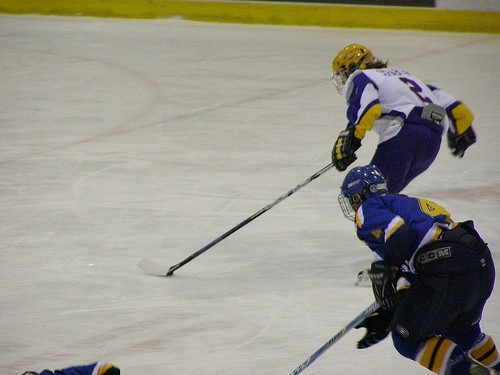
[447,124,477,158]
[353,310,395,349]
[332,126,362,171]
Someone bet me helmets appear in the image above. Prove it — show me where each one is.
[330,43,374,95]
[337,164,389,222]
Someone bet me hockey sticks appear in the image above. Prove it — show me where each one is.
[136,160,336,276]
[288,276,411,375]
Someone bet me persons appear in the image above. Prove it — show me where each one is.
[337,165,500,375]
[22,361,120,375]
[331,43,477,278]
